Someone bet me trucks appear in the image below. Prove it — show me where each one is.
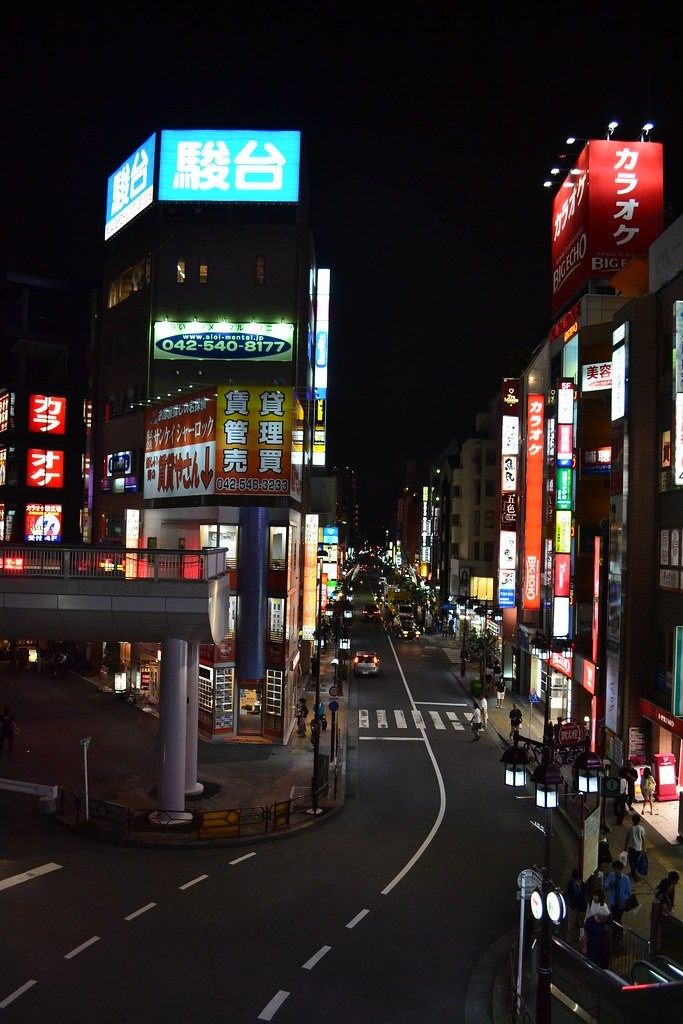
[383,584,421,640]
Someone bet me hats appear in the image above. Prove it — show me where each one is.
[596,906,609,916]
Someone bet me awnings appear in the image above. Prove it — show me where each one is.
[443,605,456,612]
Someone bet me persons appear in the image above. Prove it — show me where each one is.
[427,615,454,640]
[384,618,393,633]
[566,861,630,954]
[0,707,20,752]
[571,764,578,801]
[313,699,324,721]
[51,650,67,679]
[460,570,469,587]
[485,657,501,686]
[310,654,317,677]
[495,677,506,709]
[651,871,679,950]
[509,703,523,738]
[469,695,487,741]
[600,814,646,880]
[554,717,564,741]
[461,646,476,663]
[294,698,309,737]
[613,760,656,825]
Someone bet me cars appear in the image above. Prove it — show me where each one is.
[378,576,388,586]
[353,650,380,676]
[362,603,380,622]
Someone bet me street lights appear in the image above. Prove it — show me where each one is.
[314,549,328,729]
[497,721,607,1024]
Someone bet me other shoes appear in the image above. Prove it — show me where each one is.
[474,736,480,741]
[629,806,634,810]
[509,736,512,740]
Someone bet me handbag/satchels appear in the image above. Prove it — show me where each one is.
[643,777,655,791]
[619,851,628,865]
[298,722,306,733]
[637,851,648,875]
[624,892,639,912]
